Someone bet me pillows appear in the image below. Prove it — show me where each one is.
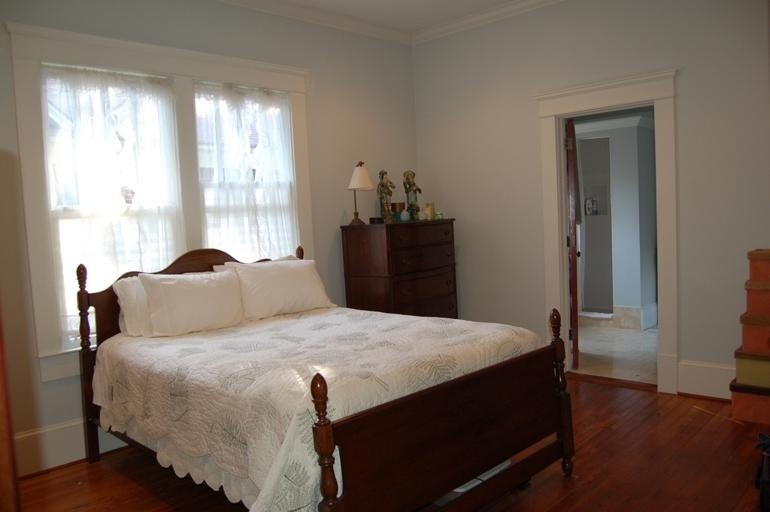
[111,255,337,338]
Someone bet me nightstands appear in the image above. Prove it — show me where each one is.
[340,218,459,319]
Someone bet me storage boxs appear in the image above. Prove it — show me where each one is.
[729,249,770,425]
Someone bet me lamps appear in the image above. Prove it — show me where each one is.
[346,160,374,226]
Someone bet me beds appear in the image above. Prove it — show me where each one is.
[77,246,566,512]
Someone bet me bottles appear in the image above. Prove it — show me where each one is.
[586,196,598,216]
